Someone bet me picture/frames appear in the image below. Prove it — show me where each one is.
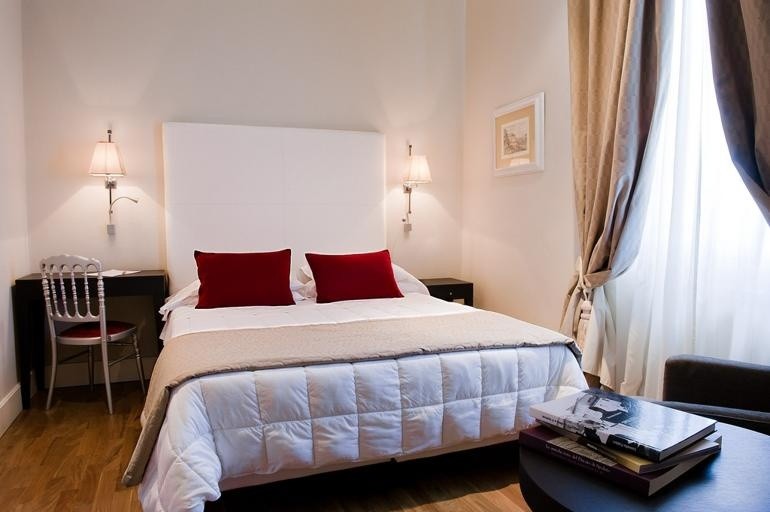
[485,89,545,179]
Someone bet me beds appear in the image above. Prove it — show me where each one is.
[164,281,569,512]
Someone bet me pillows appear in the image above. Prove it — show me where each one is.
[190,248,404,308]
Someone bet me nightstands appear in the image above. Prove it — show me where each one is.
[422,276,474,309]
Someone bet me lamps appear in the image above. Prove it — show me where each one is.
[394,141,432,224]
[87,129,141,238]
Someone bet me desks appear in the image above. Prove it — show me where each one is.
[518,417,769,512]
[13,268,170,409]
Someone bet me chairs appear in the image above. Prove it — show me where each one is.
[32,254,146,416]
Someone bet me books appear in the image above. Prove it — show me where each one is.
[518,387,723,496]
